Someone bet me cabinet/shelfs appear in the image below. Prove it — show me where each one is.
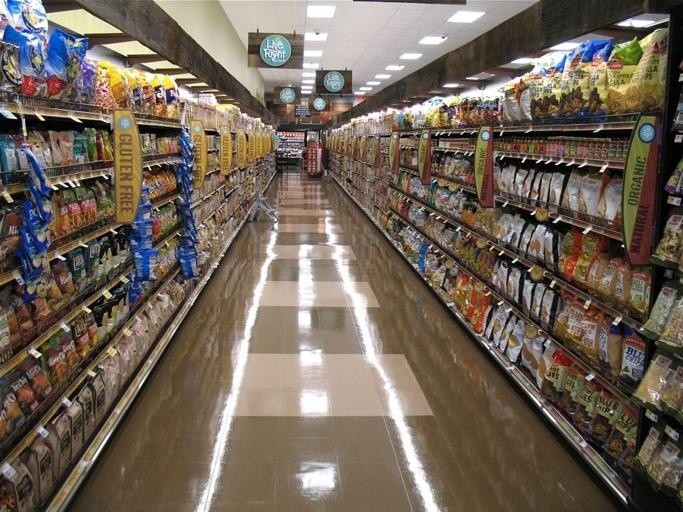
[0,0,276,512]
[323,2,683,511]
[275,131,306,165]
